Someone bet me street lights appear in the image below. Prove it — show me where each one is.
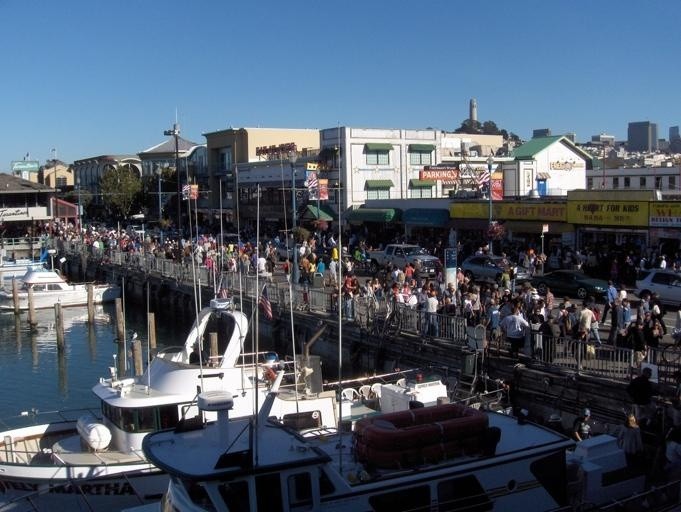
[486,154,495,254]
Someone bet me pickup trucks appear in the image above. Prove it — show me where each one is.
[368,243,440,277]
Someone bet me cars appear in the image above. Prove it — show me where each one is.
[532,270,609,300]
[636,268,681,307]
[462,255,517,285]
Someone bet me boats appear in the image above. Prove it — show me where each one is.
[1,256,122,313]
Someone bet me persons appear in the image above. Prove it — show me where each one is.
[626,367,663,430]
[623,412,646,478]
[664,433,680,466]
[572,406,594,445]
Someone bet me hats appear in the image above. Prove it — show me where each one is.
[580,406,591,417]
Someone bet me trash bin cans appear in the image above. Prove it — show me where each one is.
[462,352,475,375]
[312,273,323,288]
[530,190,541,201]
